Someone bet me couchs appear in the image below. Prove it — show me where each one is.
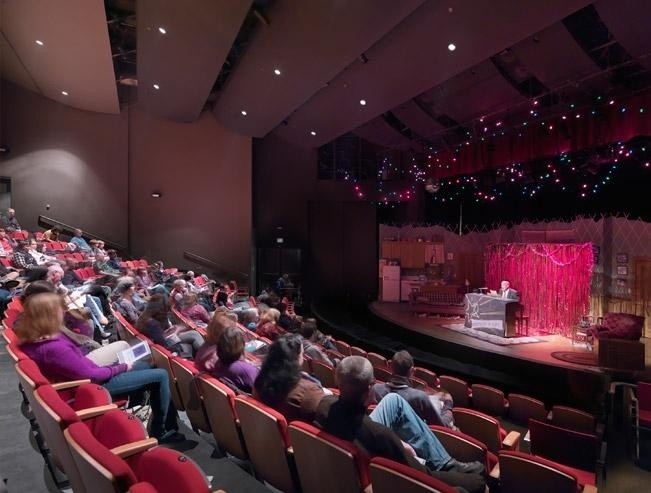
[585,311,645,354]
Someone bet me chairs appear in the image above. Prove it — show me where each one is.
[629,382,651,459]
[0,231,608,492]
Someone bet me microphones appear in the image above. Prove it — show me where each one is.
[479,287,487,290]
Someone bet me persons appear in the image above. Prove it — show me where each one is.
[2,207,489,492]
[498,280,522,300]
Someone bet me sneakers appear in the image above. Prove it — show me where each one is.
[442,458,484,474]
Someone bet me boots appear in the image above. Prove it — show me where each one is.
[149,416,185,442]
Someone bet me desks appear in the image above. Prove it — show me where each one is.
[593,336,645,370]
